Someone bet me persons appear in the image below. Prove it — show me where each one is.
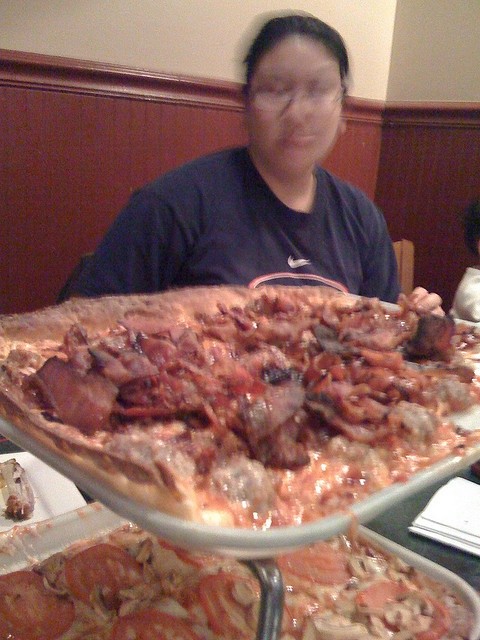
[56,9,444,320]
[448,194,479,324]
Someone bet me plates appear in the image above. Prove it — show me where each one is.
[0,452,86,532]
[0,499,480,640]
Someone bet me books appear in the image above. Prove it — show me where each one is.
[408,476,479,557]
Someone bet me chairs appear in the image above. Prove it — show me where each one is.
[392,239,415,297]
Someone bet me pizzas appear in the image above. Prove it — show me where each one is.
[0,524,480,639]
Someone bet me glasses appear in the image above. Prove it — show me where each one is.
[250,80,344,110]
